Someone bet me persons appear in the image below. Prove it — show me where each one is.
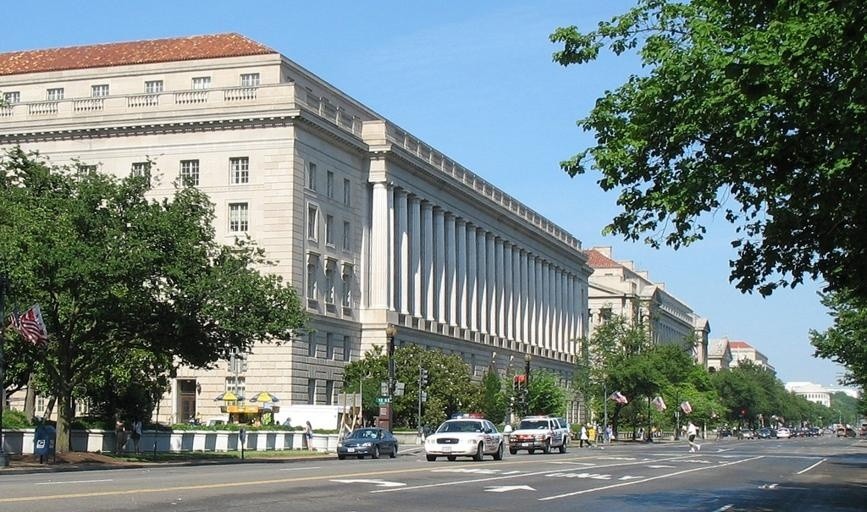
[684,420,700,453]
[650,425,658,438]
[579,421,614,450]
[283,418,291,426]
[130,415,143,456]
[638,427,645,440]
[304,420,313,452]
[115,412,129,456]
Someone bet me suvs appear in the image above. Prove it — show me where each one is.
[507,415,571,454]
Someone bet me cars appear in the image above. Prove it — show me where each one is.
[715,426,824,440]
[337,426,398,460]
[827,423,867,438]
[422,418,505,462]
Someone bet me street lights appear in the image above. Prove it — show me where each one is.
[523,353,532,414]
[385,323,396,433]
[601,370,609,427]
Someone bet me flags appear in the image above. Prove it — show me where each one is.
[611,391,628,407]
[653,397,667,412]
[8,302,47,346]
[680,401,692,415]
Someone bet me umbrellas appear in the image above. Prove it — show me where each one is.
[214,390,246,406]
[249,391,280,408]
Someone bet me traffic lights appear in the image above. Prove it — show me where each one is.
[421,368,428,386]
[423,392,427,402]
[514,377,518,390]
[519,376,524,391]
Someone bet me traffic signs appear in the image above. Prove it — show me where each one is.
[375,398,389,404]
